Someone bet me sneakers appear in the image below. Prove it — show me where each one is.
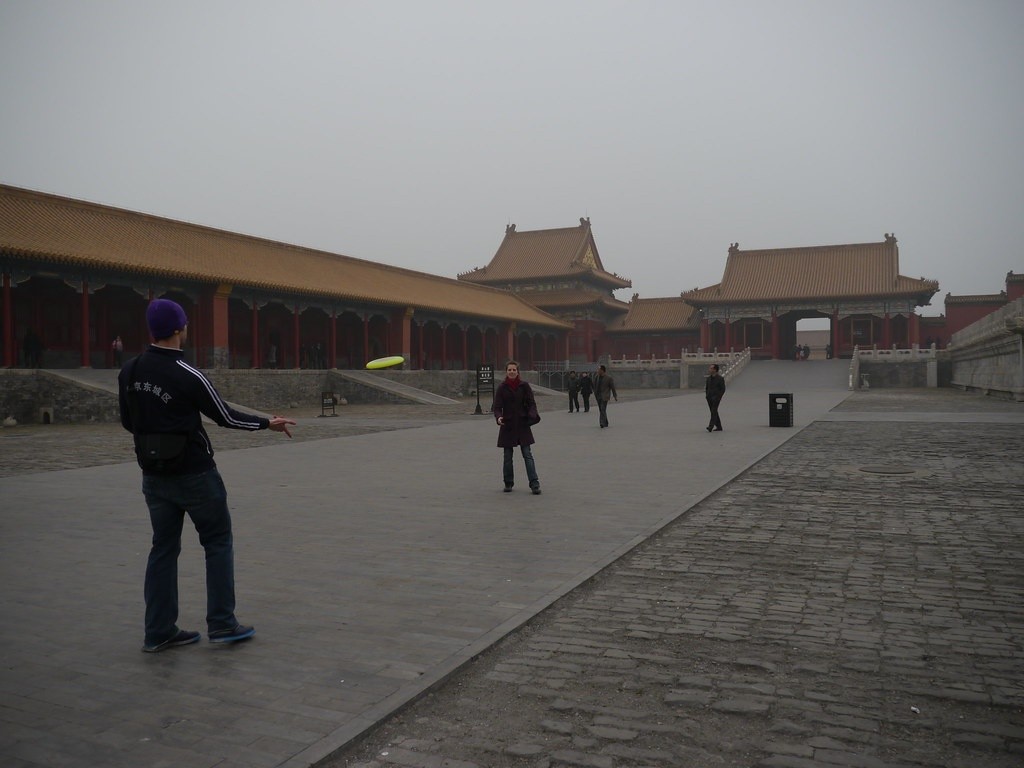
[208,624,255,643]
[142,630,201,653]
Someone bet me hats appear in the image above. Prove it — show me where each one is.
[145,299,187,340]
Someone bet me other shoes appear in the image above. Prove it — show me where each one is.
[530,484,541,494]
[504,483,513,491]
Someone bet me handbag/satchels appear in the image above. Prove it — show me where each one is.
[523,391,541,425]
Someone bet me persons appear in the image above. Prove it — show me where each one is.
[119,298,295,655]
[112,336,122,369]
[706,364,726,432]
[594,365,618,428]
[494,360,541,495]
[268,342,280,369]
[567,371,593,413]
[927,335,950,354]
[24,327,52,369]
[826,344,833,360]
[792,344,810,361]
[299,343,326,370]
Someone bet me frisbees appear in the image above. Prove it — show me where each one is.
[365,355,404,369]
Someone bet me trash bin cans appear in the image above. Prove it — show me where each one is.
[768,394,793,427]
